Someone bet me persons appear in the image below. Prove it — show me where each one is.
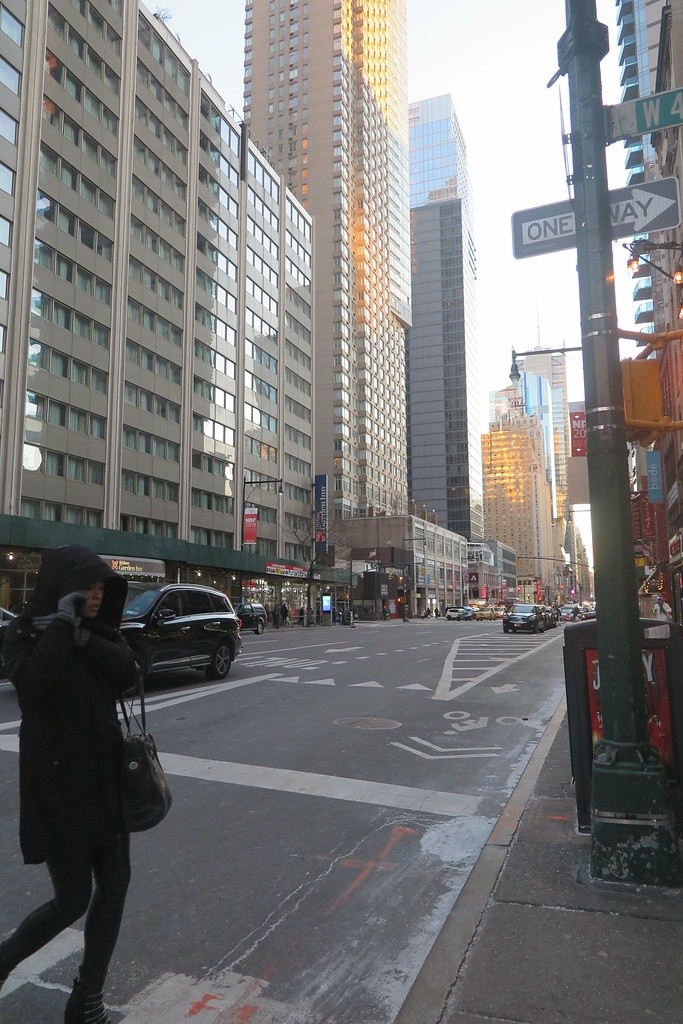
[653,595,673,623]
[0,539,141,1023]
[552,606,594,621]
[269,604,440,629]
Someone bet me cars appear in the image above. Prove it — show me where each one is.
[502,604,545,634]
[444,601,596,630]
[0,606,17,676]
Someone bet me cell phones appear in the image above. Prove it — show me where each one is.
[72,599,87,619]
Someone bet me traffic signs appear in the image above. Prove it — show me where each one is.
[612,85,683,140]
[511,175,682,260]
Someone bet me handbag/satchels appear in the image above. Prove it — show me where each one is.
[119,662,173,835]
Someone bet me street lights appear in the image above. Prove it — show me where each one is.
[400,536,427,601]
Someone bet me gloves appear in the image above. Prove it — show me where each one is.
[54,591,88,631]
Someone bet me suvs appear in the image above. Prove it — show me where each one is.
[114,581,243,696]
[230,601,268,634]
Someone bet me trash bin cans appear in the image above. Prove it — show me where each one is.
[562,619,683,833]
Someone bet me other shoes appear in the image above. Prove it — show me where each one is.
[0,941,15,992]
[64,976,113,1024]
